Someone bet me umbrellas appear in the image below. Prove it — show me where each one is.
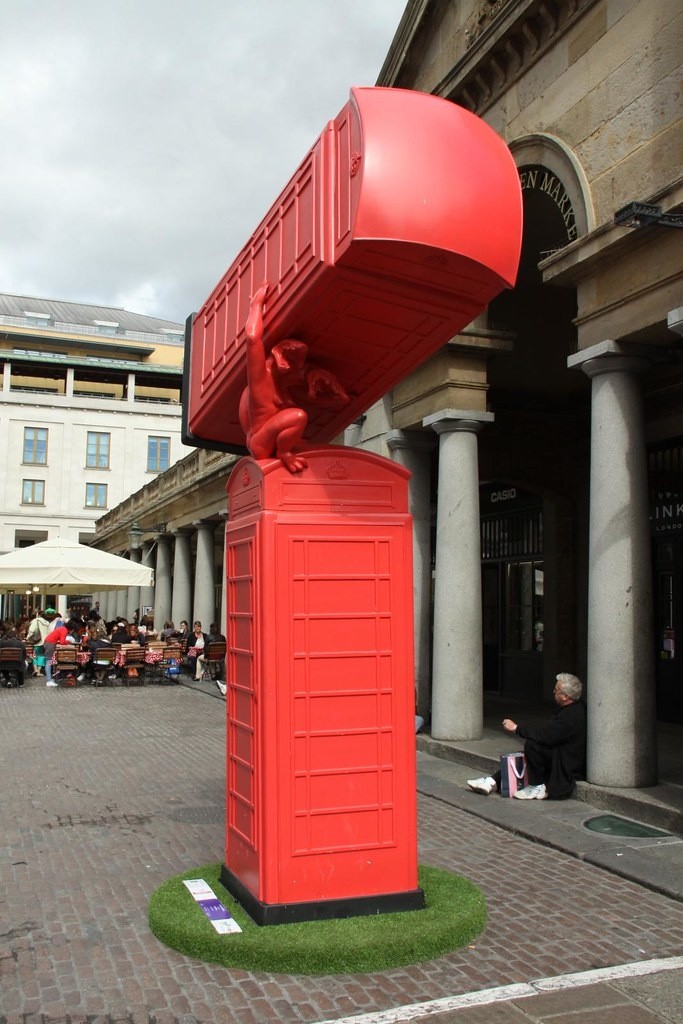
[0,536,155,620]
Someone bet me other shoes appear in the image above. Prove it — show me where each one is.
[192,678,199,681]
[216,680,227,696]
[32,671,38,676]
[46,681,58,686]
[76,675,85,682]
[36,673,44,676]
[7,682,12,688]
[20,684,25,688]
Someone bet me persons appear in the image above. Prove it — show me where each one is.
[239,280,349,474]
[0,606,227,697]
[466,672,583,800]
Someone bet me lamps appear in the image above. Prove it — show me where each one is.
[127,522,168,550]
[614,201,683,230]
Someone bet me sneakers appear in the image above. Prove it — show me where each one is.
[467,777,497,795]
[513,783,548,800]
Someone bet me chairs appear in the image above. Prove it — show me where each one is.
[0,638,226,688]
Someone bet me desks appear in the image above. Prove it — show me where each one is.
[187,647,205,657]
[30,649,94,682]
[114,650,185,682]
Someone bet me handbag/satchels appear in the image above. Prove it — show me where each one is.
[500,752,528,798]
[29,621,42,645]
